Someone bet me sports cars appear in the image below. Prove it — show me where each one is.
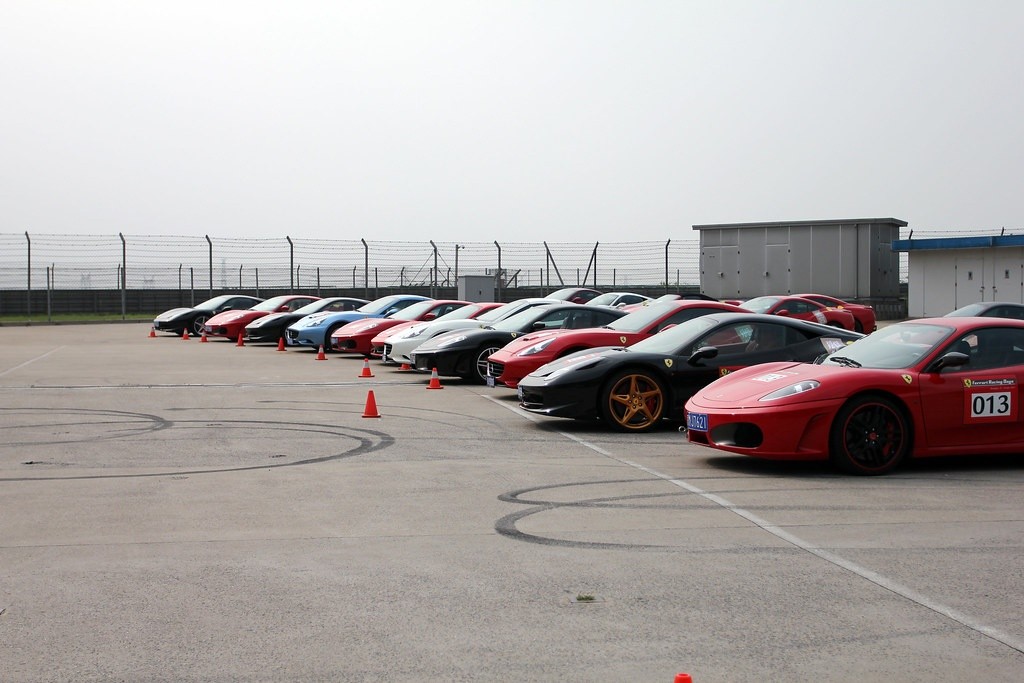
[245,296,371,345]
[487,299,757,388]
[542,286,878,334]
[370,301,511,359]
[410,304,629,385]
[382,298,579,371]
[287,294,438,354]
[203,294,324,342]
[331,301,476,359]
[684,317,1024,476]
[517,312,868,435]
[152,294,266,337]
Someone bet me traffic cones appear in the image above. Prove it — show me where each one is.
[148,326,158,339]
[314,343,328,360]
[180,327,191,340]
[398,363,412,371]
[425,367,443,389]
[200,330,210,343]
[276,337,288,351]
[236,332,247,347]
[362,390,381,418]
[358,357,376,378]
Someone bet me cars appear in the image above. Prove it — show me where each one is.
[943,300,1024,321]
